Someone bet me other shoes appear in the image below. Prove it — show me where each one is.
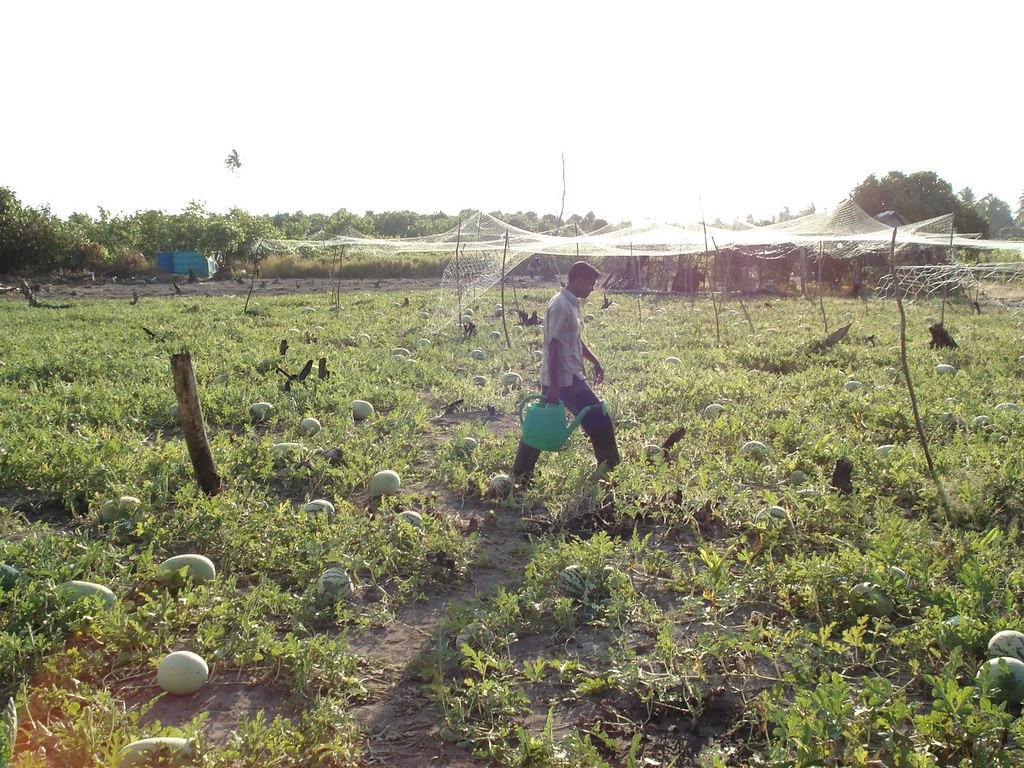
[512,471,537,488]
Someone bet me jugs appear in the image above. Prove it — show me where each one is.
[517,394,608,453]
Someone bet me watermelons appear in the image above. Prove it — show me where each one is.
[0,302,1024,768]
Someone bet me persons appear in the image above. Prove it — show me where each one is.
[510,260,620,475]
[254,260,260,278]
